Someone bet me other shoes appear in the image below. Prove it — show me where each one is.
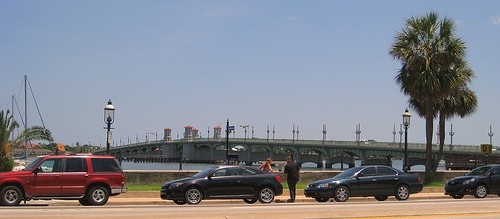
[287,199,295,202]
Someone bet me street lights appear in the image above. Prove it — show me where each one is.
[402,107,411,173]
[103,98,116,154]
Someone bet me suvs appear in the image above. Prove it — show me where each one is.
[0,151,129,206]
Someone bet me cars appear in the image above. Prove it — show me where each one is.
[159,165,284,206]
[304,164,424,202]
[444,163,500,199]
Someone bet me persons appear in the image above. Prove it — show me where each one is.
[259,158,273,172]
[284,156,300,202]
[52,144,67,172]
